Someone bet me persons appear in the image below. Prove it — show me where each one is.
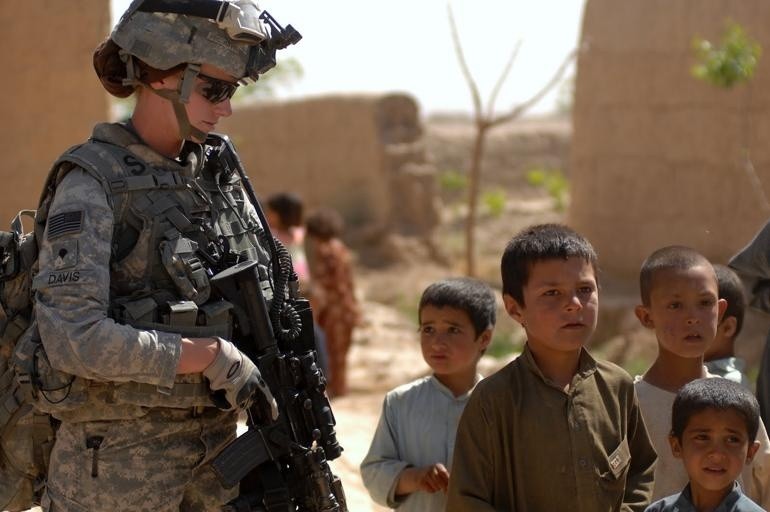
[299,205,363,396]
[27,0,307,510]
[356,275,499,511]
[640,377,770,511]
[633,244,770,511]
[706,263,749,387]
[443,221,660,511]
[259,185,312,293]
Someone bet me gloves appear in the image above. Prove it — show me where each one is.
[199,332,280,426]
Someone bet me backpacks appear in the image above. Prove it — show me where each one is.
[0,137,148,512]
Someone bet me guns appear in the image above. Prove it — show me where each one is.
[208,260,347,511]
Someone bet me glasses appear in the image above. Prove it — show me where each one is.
[174,70,241,103]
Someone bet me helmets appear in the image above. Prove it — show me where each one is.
[107,0,280,84]
